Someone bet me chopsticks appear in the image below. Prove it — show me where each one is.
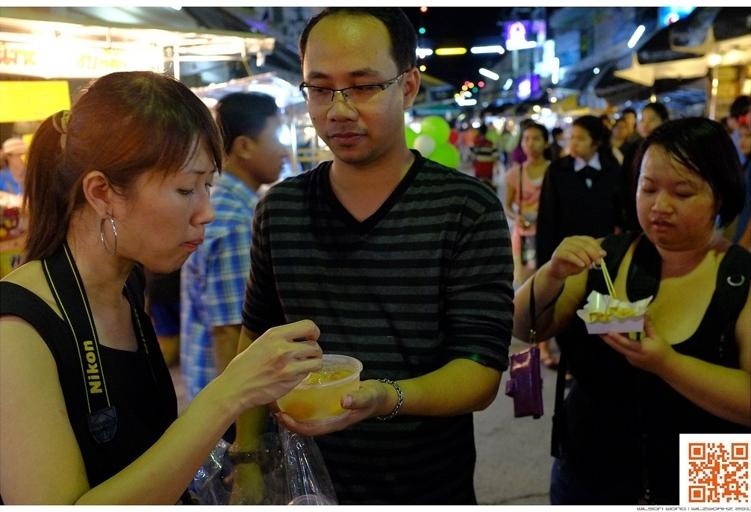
[600,257,617,300]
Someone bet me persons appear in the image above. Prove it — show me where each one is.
[514,116,751,505]
[0,69,324,505]
[180,93,287,505]
[0,138,29,195]
[231,6,513,505]
[443,94,751,366]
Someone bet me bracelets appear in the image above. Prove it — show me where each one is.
[378,377,404,423]
[224,451,267,465]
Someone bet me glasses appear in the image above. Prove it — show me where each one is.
[299,69,408,107]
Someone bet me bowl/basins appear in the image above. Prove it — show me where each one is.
[276,354,363,422]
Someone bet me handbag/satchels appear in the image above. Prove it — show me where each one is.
[521,235,537,265]
